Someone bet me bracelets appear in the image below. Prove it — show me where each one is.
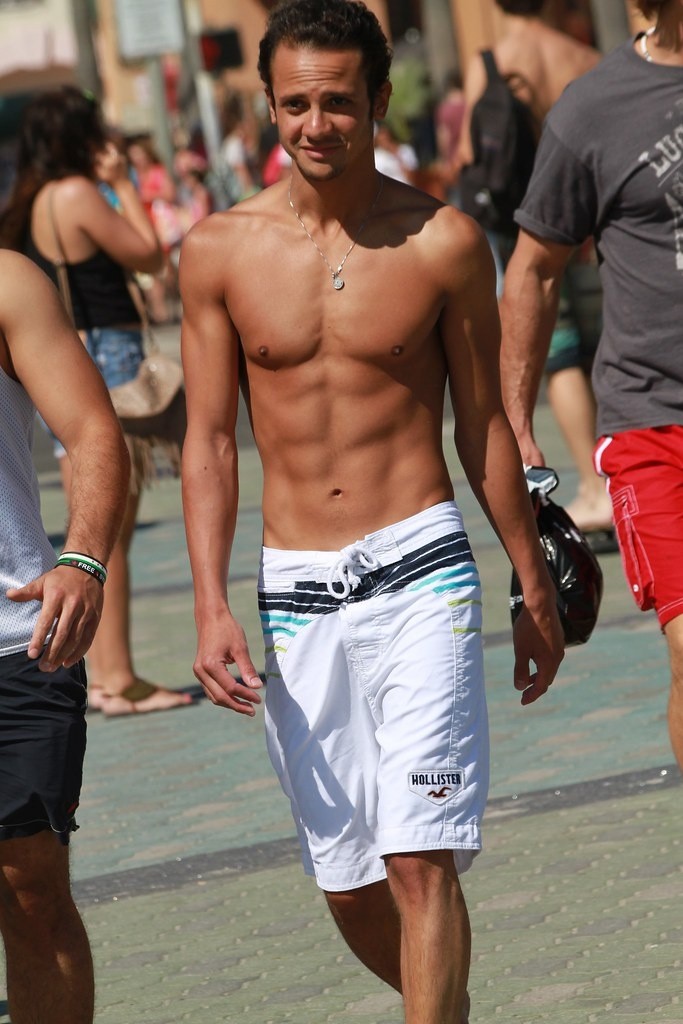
[53,552,107,586]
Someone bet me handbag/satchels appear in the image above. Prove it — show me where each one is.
[104,354,188,494]
[508,462,603,648]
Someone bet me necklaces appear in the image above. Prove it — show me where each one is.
[640,27,656,62]
[288,173,384,290]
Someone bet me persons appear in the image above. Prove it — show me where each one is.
[181,0,565,1024]
[500,0,683,772]
[0,86,193,718]
[458,0,620,557]
[434,68,465,159]
[0,249,132,1024]
[374,120,422,189]
[100,103,292,321]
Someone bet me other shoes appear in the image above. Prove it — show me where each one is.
[85,684,107,711]
[569,526,620,554]
[101,677,195,718]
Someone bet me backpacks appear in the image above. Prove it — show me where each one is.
[442,49,542,240]
